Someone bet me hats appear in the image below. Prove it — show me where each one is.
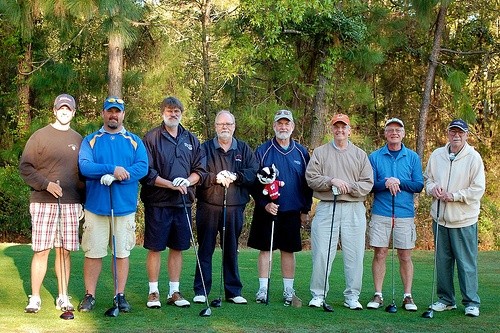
[104,96,124,112]
[385,118,404,127]
[54,94,76,111]
[274,110,294,123]
[332,114,350,126]
[448,119,468,132]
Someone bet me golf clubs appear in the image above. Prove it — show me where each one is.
[56,179,74,320]
[103,184,119,317]
[264,214,275,305]
[210,186,228,307]
[323,195,337,312]
[385,195,397,312]
[179,192,212,317]
[422,200,440,319]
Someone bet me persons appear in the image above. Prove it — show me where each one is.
[192,110,260,304]
[366,118,424,312]
[305,113,374,310]
[424,120,485,318]
[139,96,210,309]
[77,96,149,313]
[247,109,314,307]
[19,94,86,313]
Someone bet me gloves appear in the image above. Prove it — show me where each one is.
[217,170,237,188]
[101,174,117,186]
[172,178,190,187]
[332,185,341,195]
[79,209,84,221]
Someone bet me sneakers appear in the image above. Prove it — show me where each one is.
[256,289,267,303]
[344,298,363,310]
[24,295,41,313]
[465,306,479,316]
[193,294,206,303]
[168,291,190,307]
[147,292,161,308]
[226,295,247,304]
[78,294,95,312]
[55,295,75,311]
[403,295,417,310]
[113,293,130,312]
[309,297,323,307]
[429,301,457,312]
[283,290,295,306]
[367,293,383,308]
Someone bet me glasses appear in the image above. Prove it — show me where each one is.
[276,111,290,115]
[385,128,404,133]
[215,123,235,127]
[107,98,124,104]
[449,129,468,137]
